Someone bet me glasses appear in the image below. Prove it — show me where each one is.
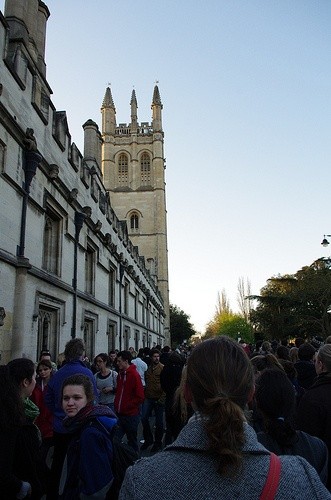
[96,359,104,363]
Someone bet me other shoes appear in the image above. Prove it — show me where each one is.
[149,441,163,454]
[140,440,153,450]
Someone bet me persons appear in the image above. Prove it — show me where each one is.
[42,373,115,500]
[121,336,331,500]
[32,354,63,453]
[35,335,331,500]
[0,358,45,500]
[48,338,98,456]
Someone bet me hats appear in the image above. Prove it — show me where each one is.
[311,335,325,349]
